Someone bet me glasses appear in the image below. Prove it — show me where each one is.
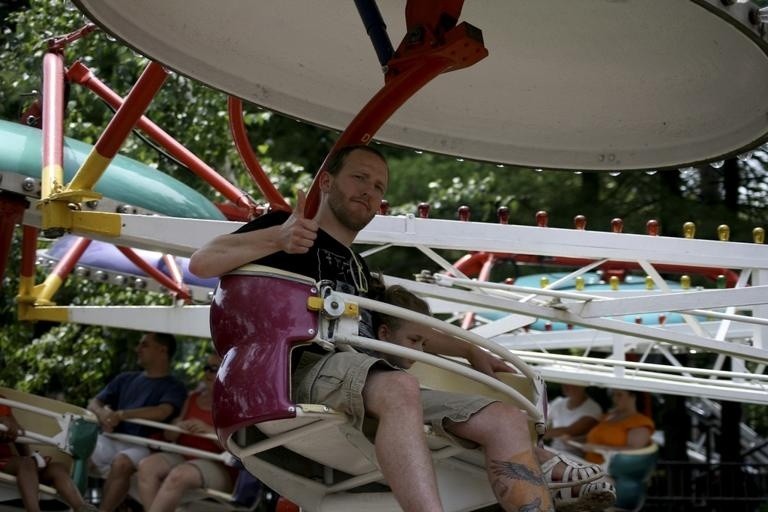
[202,363,218,373]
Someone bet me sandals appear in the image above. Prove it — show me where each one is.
[550,481,616,512]
[539,453,605,489]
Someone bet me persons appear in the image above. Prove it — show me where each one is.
[188,143,556,512]
[543,384,603,456]
[0,395,99,512]
[53,331,188,512]
[136,349,239,512]
[372,285,618,512]
[584,389,655,464]
